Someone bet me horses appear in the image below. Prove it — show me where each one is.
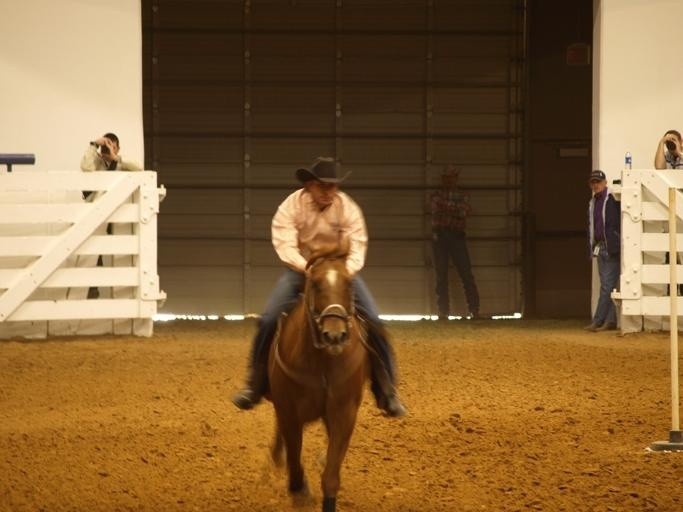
[261,234,370,511]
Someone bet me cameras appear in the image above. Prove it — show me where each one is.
[101,145,110,155]
[666,140,676,151]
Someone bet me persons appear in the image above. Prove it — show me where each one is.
[584,167,621,332]
[653,129,683,170]
[77,132,143,203]
[428,163,482,320]
[233,154,408,419]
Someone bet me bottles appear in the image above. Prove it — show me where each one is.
[625,151,632,171]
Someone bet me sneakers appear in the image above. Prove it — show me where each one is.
[583,319,617,331]
[234,388,260,410]
[377,396,405,418]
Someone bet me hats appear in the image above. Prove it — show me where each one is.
[295,157,352,184]
[588,170,606,182]
[439,162,462,176]
